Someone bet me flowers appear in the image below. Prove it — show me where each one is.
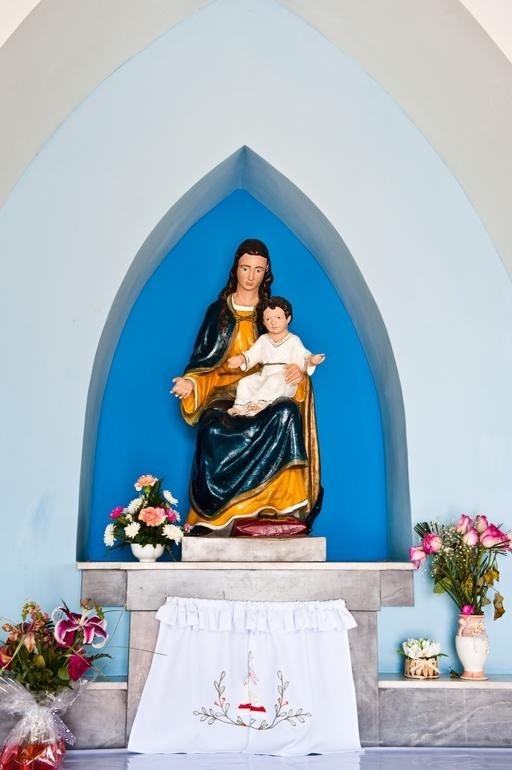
[395,639,448,661]
[0,592,172,770]
[101,474,188,562]
[406,513,512,622]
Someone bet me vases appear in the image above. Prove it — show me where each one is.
[454,612,491,681]
[130,542,167,562]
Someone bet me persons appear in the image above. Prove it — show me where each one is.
[169,238,324,537]
[225,295,326,417]
